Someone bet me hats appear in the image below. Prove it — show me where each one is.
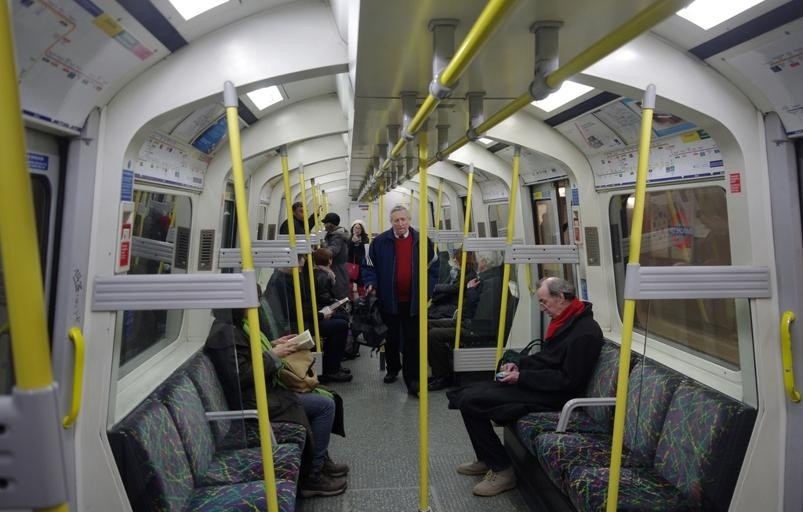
[321,213,340,226]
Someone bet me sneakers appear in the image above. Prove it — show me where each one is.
[406,377,421,395]
[471,464,518,498]
[455,457,489,476]
[300,472,348,500]
[324,367,354,383]
[322,449,352,478]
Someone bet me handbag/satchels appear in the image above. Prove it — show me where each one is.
[275,349,319,394]
[494,338,545,384]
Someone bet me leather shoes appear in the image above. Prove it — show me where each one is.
[428,372,465,393]
[383,363,403,383]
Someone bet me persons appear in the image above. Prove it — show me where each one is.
[322,214,350,308]
[348,223,369,271]
[427,246,503,387]
[311,248,345,311]
[280,201,321,235]
[362,206,441,395]
[275,254,352,383]
[445,277,605,498]
[203,282,350,499]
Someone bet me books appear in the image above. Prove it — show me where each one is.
[286,328,315,350]
[328,296,350,311]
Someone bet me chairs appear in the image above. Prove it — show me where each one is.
[107,291,320,512]
[459,290,757,512]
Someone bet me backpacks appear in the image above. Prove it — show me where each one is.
[350,293,391,348]
